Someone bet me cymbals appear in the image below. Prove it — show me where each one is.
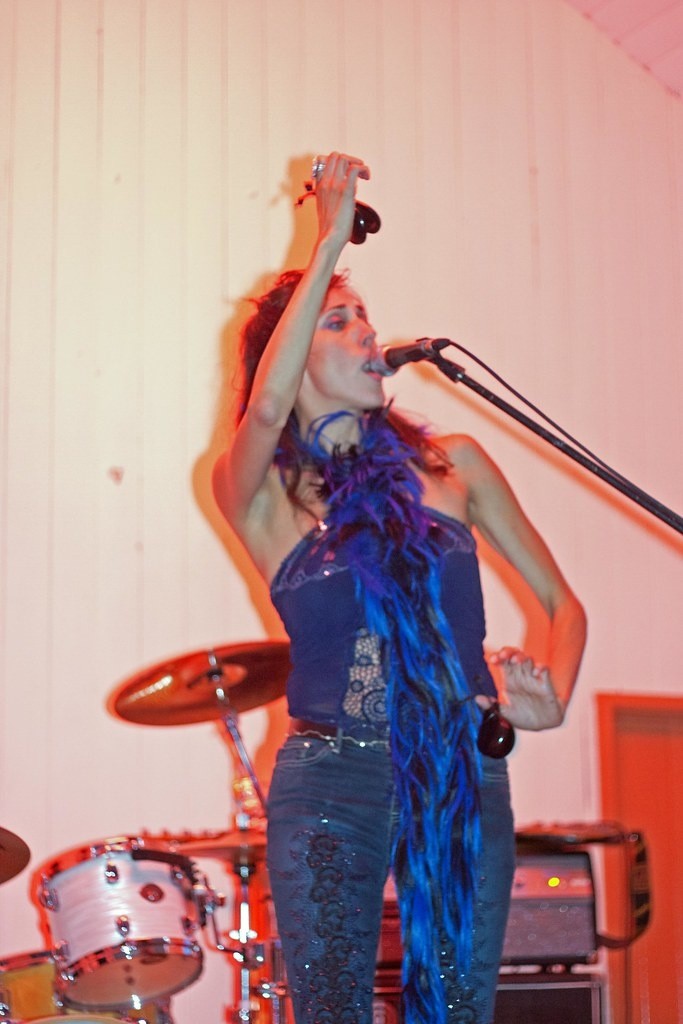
[164,830,268,862]
[0,827,32,883]
[113,640,292,726]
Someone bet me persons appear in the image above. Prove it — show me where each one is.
[211,151,587,1024]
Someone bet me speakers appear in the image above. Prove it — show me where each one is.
[371,972,610,1024]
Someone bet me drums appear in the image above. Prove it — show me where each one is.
[238,933,295,1024]
[0,835,206,1024]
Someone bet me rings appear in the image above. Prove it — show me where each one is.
[312,164,326,177]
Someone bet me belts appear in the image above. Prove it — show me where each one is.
[286,717,338,742]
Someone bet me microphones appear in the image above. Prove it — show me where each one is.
[369,337,452,376]
